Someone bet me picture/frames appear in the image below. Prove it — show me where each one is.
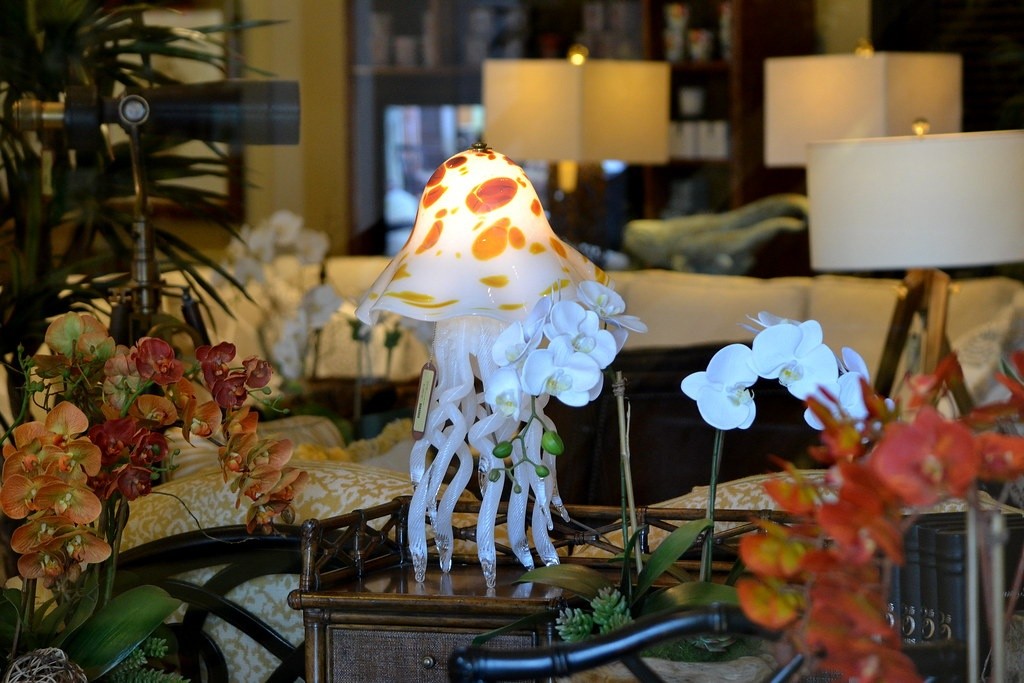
[0,0,245,224]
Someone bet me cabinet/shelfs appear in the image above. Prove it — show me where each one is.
[346,0,815,277]
[289,494,816,683]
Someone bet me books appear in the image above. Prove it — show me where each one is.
[881,510,1024,683]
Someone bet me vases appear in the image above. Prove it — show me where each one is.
[340,403,414,441]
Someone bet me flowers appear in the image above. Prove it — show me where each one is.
[482,280,898,663]
[212,207,402,437]
[0,311,308,683]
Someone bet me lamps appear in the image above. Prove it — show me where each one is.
[482,45,671,244]
[806,123,1023,437]
[764,35,961,166]
